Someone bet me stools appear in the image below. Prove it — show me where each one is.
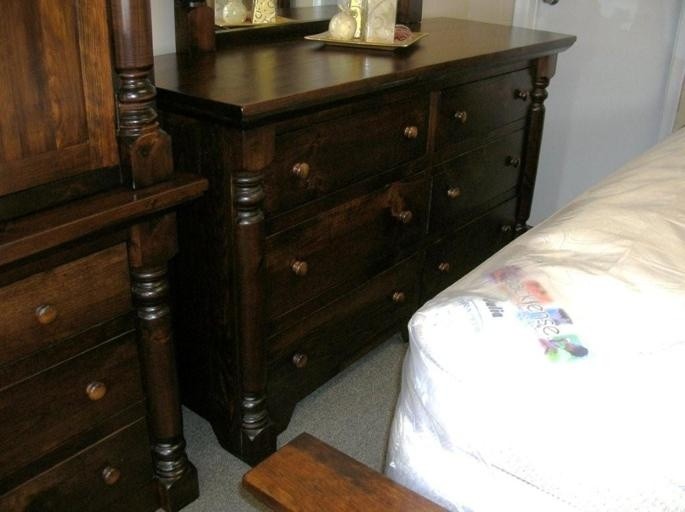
[243,431,453,512]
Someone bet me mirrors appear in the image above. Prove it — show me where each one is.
[174,0,423,55]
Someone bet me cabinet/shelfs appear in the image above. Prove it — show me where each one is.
[1,0,212,511]
[154,16,576,469]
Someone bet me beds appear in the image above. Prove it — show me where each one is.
[382,125,685,512]
